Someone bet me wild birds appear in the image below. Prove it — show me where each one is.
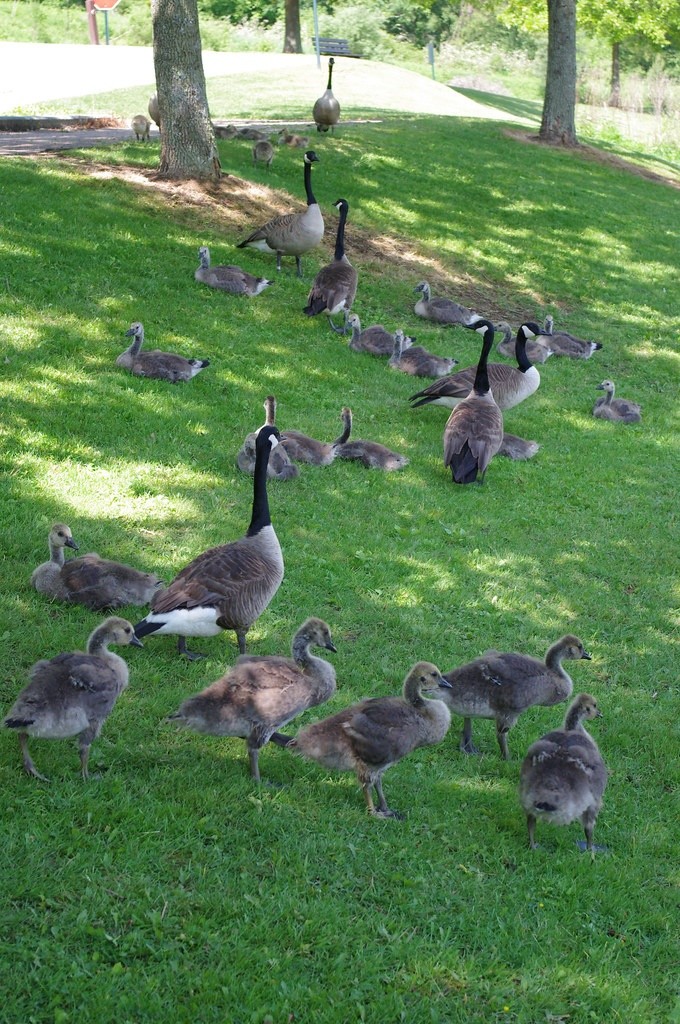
[1,616,144,784]
[346,314,459,376]
[285,660,453,821]
[236,151,325,277]
[164,616,338,779]
[313,58,340,135]
[443,320,539,486]
[408,320,550,412]
[334,407,410,469]
[301,197,357,333]
[194,247,276,297]
[237,396,334,482]
[518,692,608,865]
[412,282,483,325]
[30,522,164,611]
[130,96,309,168]
[116,322,210,383]
[421,634,593,762]
[135,426,287,661]
[493,315,642,424]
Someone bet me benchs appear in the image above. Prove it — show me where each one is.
[313,38,363,58]
